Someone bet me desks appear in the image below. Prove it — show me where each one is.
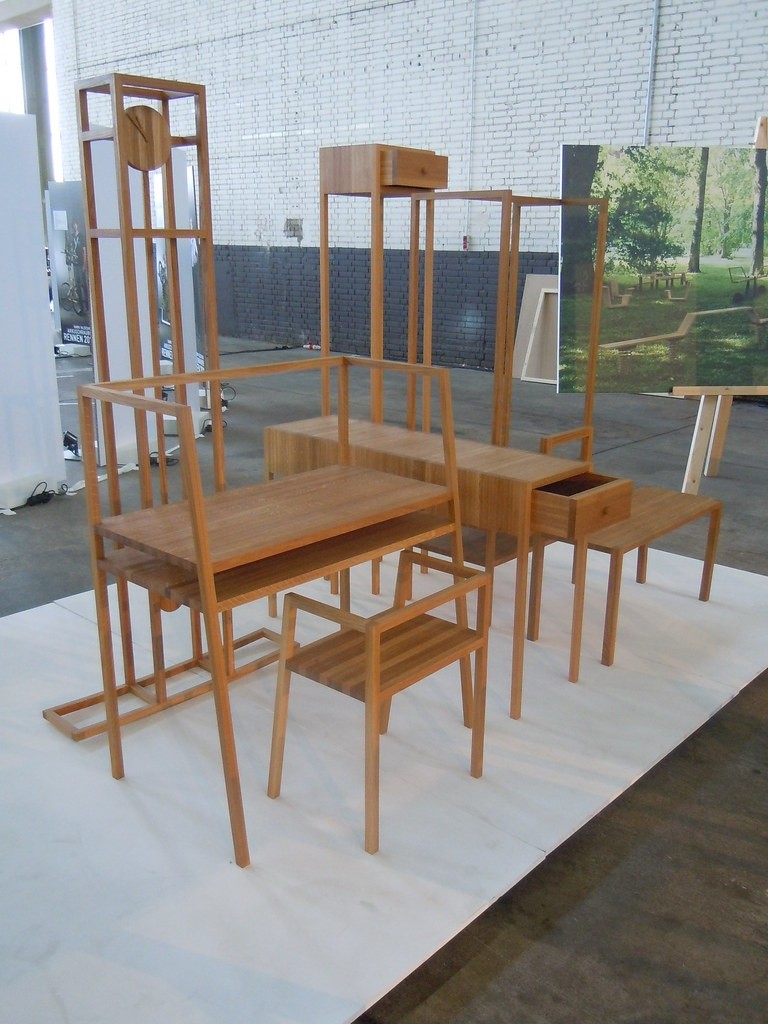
[527,486,724,666]
[255,415,632,720]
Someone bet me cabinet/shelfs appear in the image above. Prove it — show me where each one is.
[40,72,301,741]
[317,145,449,608]
[77,356,488,867]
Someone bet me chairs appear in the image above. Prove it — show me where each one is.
[266,551,493,854]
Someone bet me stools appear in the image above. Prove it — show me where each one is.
[670,386,767,497]
